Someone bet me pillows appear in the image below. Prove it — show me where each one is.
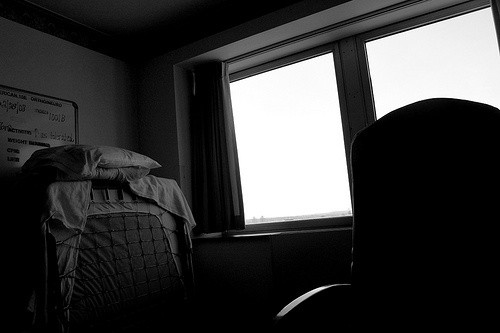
[23,163,151,183]
[23,144,163,173]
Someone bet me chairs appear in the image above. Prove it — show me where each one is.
[266,98,500,333]
